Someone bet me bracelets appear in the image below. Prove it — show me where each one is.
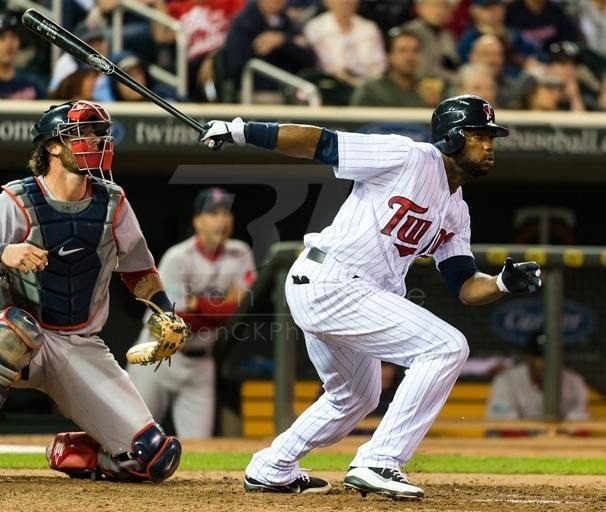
[149,290,172,315]
[244,120,279,152]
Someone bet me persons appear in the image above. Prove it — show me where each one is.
[199,95,544,499]
[0,102,187,484]
[125,188,257,437]
[0,0,606,114]
[486,333,591,438]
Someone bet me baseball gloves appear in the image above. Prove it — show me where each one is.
[125,311,193,365]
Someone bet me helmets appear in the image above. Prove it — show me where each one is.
[431,95,508,155]
[31,100,118,188]
[196,189,234,212]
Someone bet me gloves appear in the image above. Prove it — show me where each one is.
[199,121,279,149]
[497,258,542,294]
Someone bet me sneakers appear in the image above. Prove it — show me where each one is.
[344,466,424,499]
[245,472,331,493]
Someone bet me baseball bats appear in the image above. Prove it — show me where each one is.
[22,7,225,152]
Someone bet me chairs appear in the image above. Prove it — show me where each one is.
[198,40,357,106]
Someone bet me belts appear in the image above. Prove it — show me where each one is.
[306,248,327,267]
